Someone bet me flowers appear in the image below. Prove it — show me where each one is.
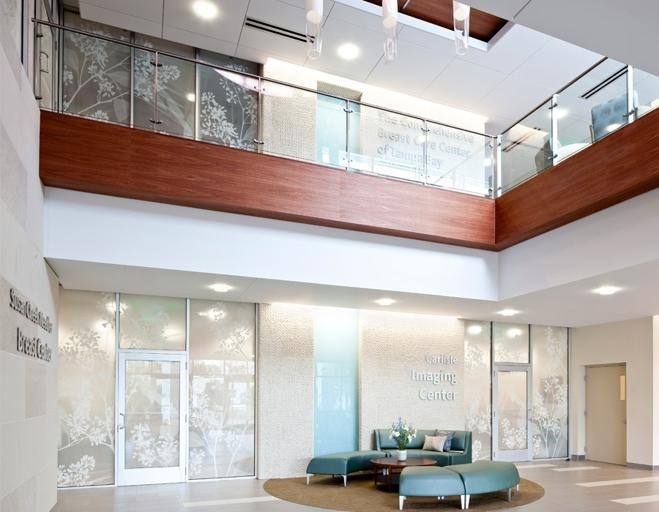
[387,415,418,450]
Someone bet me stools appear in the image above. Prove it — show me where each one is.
[398,458,522,509]
[306,449,385,487]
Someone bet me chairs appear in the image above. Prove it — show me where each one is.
[588,89,652,145]
[534,139,564,175]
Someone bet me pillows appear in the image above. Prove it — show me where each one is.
[423,429,455,452]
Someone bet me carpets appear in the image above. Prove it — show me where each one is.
[260,476,543,512]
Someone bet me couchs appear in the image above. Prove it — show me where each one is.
[375,427,474,466]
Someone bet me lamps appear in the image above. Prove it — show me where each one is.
[305,0,473,63]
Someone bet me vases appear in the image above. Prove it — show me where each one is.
[395,450,407,461]
